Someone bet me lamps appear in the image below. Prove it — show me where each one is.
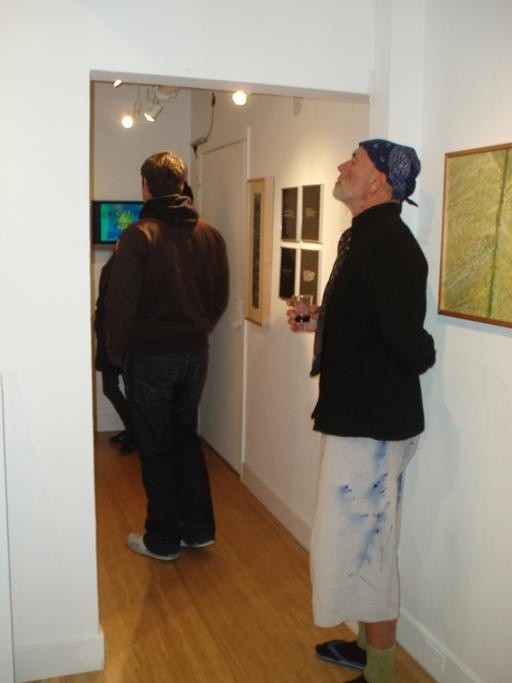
[113,80,252,129]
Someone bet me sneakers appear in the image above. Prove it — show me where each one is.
[183,537,219,550]
[110,429,139,457]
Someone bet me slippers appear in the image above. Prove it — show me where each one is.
[126,532,182,563]
[314,638,371,670]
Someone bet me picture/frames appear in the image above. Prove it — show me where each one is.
[278,183,325,308]
[243,177,273,326]
[437,141,512,328]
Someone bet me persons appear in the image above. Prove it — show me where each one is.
[101,147,231,562]
[92,239,137,455]
[285,140,438,682]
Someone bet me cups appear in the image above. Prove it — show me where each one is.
[292,294,311,324]
